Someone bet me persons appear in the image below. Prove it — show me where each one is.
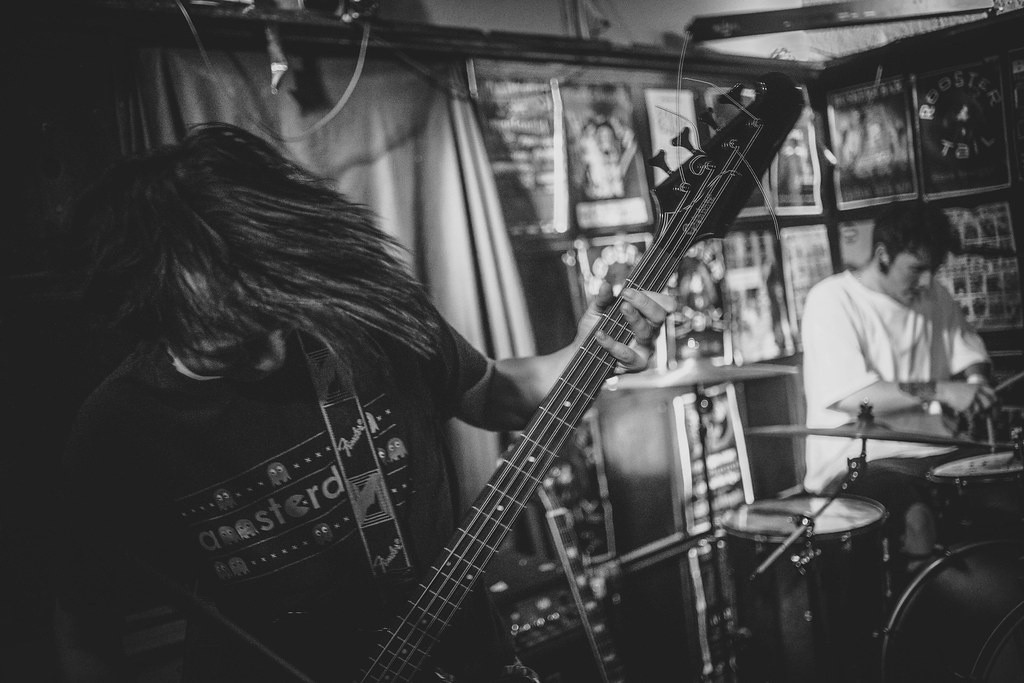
[55,124,679,682]
[802,202,1002,561]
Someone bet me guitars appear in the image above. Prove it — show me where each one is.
[346,68,806,683]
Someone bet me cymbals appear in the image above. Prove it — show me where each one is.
[601,356,799,391]
[741,418,996,449]
[930,450,1022,478]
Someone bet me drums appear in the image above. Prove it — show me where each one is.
[719,489,891,645]
[873,531,1024,683]
